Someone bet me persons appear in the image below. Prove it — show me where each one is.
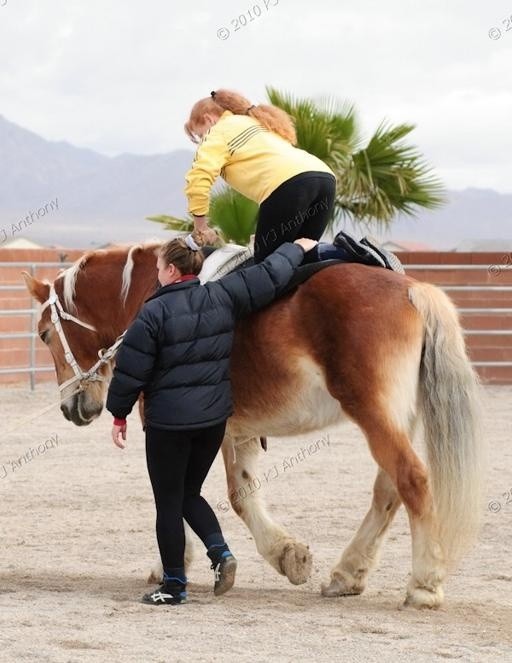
[184,88,408,275]
[104,230,320,607]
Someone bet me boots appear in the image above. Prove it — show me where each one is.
[317,231,386,269]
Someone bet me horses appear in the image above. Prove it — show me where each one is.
[17,241,488,612]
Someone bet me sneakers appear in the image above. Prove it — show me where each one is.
[140,576,187,605]
[207,543,237,595]
[357,234,406,275]
